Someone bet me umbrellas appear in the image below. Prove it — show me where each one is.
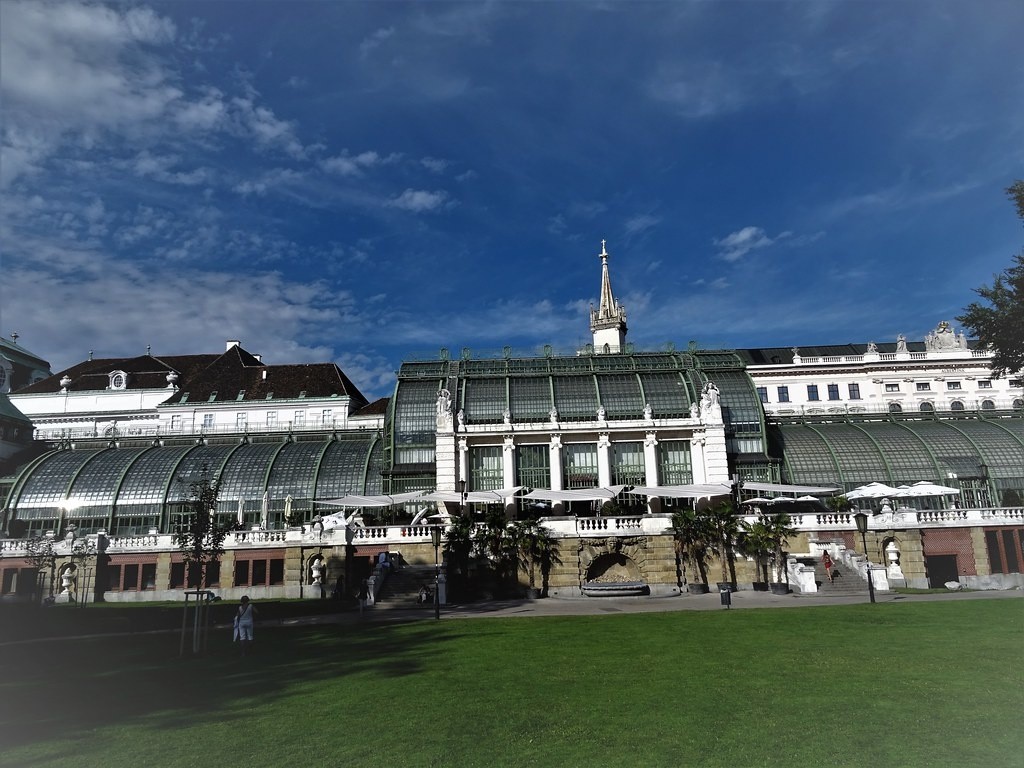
[237,494,245,525]
[743,497,773,503]
[771,496,796,501]
[796,495,819,501]
[261,491,268,530]
[284,495,294,530]
[841,481,959,500]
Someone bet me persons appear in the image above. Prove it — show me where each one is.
[334,575,345,594]
[416,585,433,608]
[549,407,558,422]
[458,408,465,425]
[643,404,652,419]
[699,381,721,407]
[502,408,510,423]
[821,550,836,582]
[436,389,451,413]
[596,405,605,420]
[357,579,371,609]
[380,551,400,577]
[688,402,698,417]
[236,596,258,656]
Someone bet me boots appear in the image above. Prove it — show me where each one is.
[240,640,247,656]
[247,640,254,654]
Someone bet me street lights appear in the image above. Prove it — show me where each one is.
[458,478,466,514]
[979,462,992,508]
[429,526,442,621]
[853,512,875,603]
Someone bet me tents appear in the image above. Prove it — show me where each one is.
[307,485,732,507]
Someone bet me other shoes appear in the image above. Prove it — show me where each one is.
[232,643,236,648]
[236,640,240,647]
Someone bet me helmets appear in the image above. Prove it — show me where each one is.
[241,596,249,603]
